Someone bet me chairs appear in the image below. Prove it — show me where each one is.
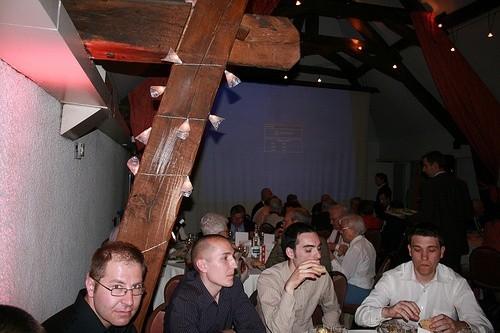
[144,232,435,333]
[464,246,500,322]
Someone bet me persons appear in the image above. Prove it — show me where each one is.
[201,152,500,275]
[162,234,266,333]
[41,242,148,333]
[257,222,341,333]
[354,225,494,333]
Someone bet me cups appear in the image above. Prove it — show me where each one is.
[306,323,348,333]
[238,243,249,260]
[376,317,418,333]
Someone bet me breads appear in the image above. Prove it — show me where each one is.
[312,265,327,274]
[420,318,434,331]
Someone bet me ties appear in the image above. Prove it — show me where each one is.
[331,231,342,255]
[233,226,238,236]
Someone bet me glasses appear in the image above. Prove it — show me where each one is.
[340,227,348,233]
[282,219,287,225]
[219,230,229,236]
[92,277,146,296]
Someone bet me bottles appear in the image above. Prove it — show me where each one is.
[250,225,261,261]
